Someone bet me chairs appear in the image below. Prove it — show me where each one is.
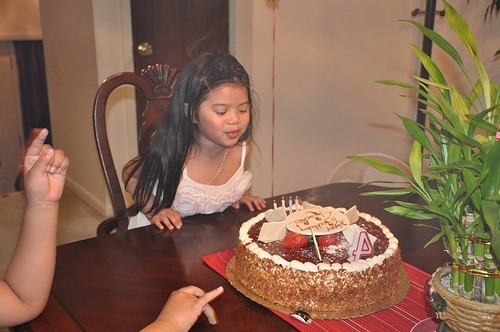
[91,61,188,236]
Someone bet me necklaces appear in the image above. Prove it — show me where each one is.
[204,149,227,185]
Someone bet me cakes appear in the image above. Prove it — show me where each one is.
[234,201,400,311]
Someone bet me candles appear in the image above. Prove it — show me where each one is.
[281,195,287,216]
[295,196,299,211]
[288,195,293,214]
[273,199,278,209]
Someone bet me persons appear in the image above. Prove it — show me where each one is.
[141,285,223,332]
[0,128,70,328]
[121,52,266,230]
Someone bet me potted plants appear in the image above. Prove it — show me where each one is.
[345,0,500,332]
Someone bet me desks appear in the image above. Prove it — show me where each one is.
[8,181,448,332]
